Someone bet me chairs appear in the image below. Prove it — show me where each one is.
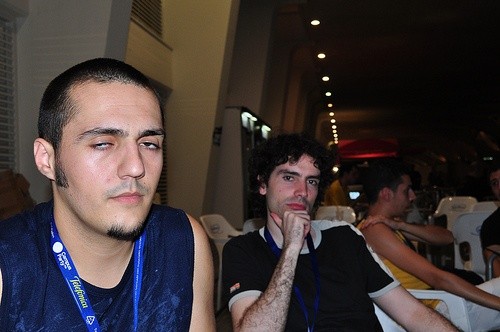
[197,195,500,332]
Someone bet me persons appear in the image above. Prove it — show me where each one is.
[434,162,499,277]
[355,155,500,332]
[0,58,215,332]
[222,133,466,332]
[324,162,359,208]
